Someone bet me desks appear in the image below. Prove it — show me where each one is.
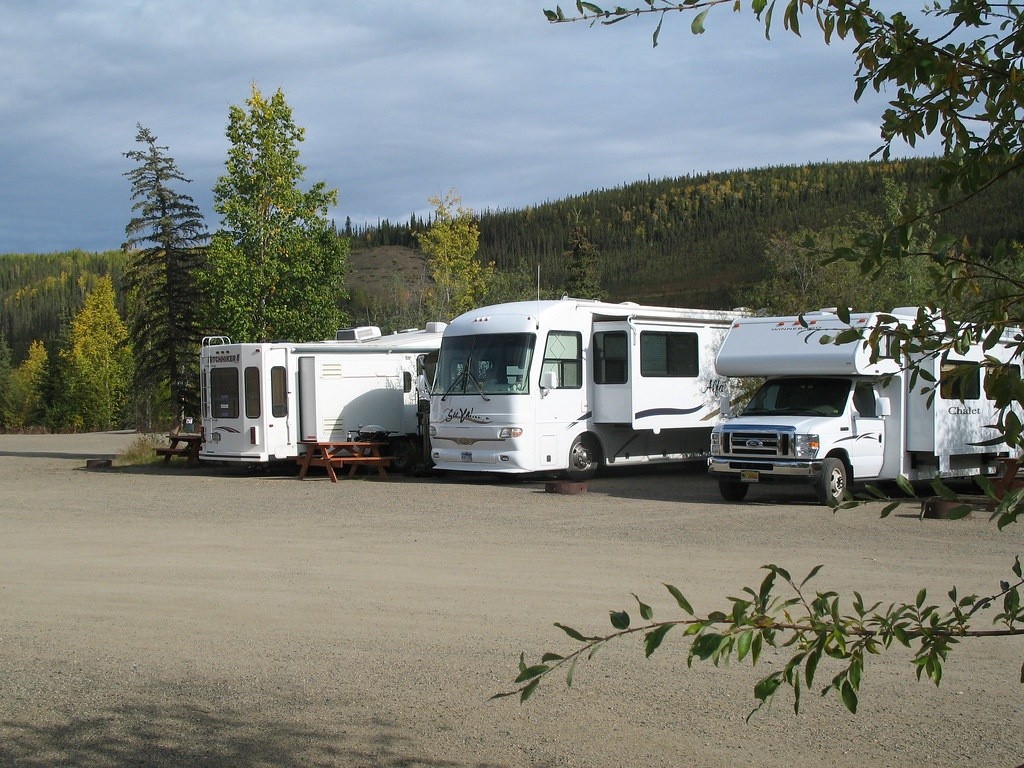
[159,435,203,470]
[297,441,389,483]
[986,457,1024,512]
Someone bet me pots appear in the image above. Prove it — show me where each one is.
[360,425,386,440]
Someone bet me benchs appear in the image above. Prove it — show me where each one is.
[985,476,1024,512]
[153,447,202,470]
[287,456,401,482]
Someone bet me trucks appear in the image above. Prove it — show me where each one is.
[195,321,531,472]
[705,306,1024,506]
[416,289,784,482]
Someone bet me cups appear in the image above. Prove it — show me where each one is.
[308,435,315,439]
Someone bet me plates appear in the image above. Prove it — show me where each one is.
[301,439,317,442]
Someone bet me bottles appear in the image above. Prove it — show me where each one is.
[346,432,352,442]
